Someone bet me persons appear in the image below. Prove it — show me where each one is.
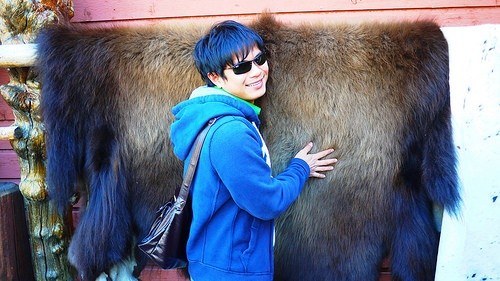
[168,19,338,281]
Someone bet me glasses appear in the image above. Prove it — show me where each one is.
[221,50,268,76]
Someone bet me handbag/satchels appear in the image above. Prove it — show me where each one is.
[137,190,192,269]
